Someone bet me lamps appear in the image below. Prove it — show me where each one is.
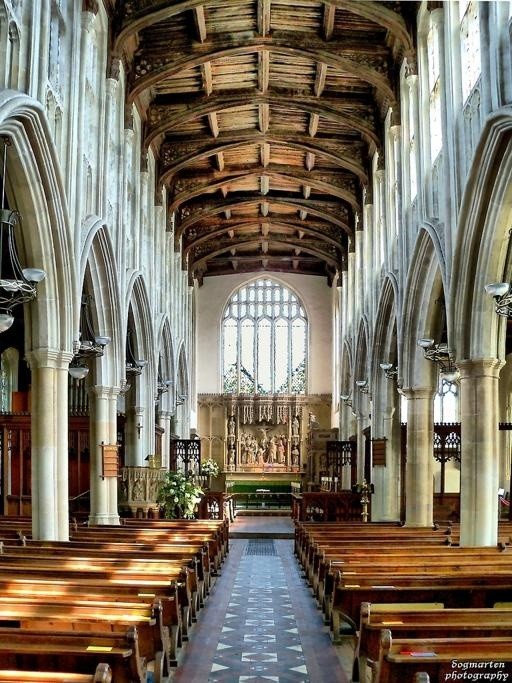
[126,328,148,392]
[380,361,397,380]
[353,377,369,397]
[156,372,173,407]
[340,392,349,407]
[416,337,451,371]
[68,293,112,385]
[486,281,512,317]
[178,391,186,405]
[1,208,47,333]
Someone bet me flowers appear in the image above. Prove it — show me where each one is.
[201,459,217,476]
[160,471,204,517]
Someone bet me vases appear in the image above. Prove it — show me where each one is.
[208,476,212,489]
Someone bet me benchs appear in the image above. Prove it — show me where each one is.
[1,515,229,682]
[294,521,512,682]
